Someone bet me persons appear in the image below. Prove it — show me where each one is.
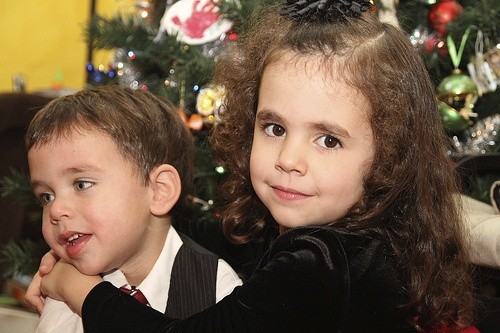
[24,0,477,333]
[25,85,245,333]
